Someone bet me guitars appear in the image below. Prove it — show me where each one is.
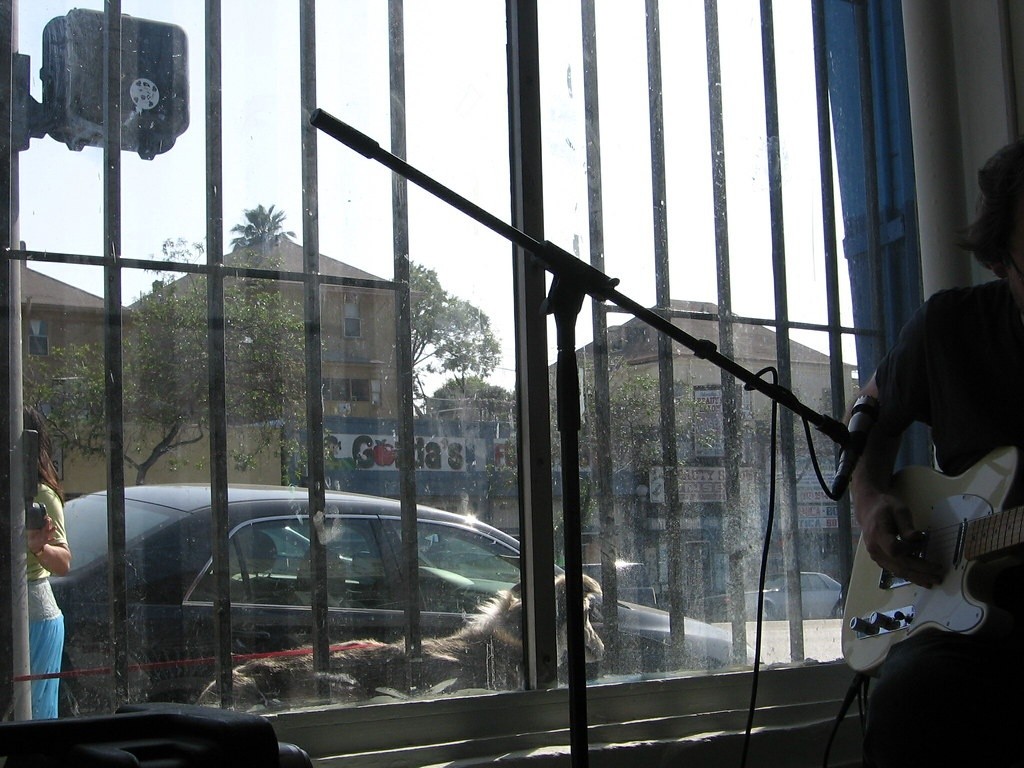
[839,443,1024,678]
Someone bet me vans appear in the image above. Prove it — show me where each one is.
[581,560,660,609]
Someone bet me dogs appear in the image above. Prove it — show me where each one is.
[193,570,606,712]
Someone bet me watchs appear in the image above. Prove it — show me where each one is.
[32,545,44,558]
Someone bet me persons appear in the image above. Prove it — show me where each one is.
[22,404,74,723]
[840,136,1024,768]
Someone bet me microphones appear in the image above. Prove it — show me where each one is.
[830,396,882,501]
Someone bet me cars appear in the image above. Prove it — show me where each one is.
[743,571,842,621]
[50,482,771,715]
